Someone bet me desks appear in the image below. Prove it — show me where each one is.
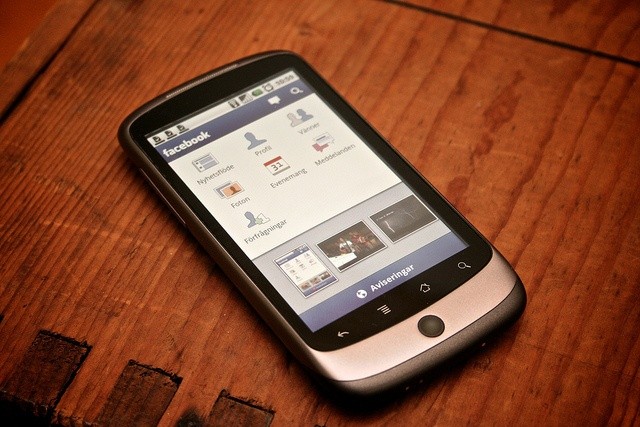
[1,0,638,424]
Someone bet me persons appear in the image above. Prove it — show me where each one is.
[335,230,372,254]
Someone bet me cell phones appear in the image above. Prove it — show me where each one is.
[117,49,528,401]
